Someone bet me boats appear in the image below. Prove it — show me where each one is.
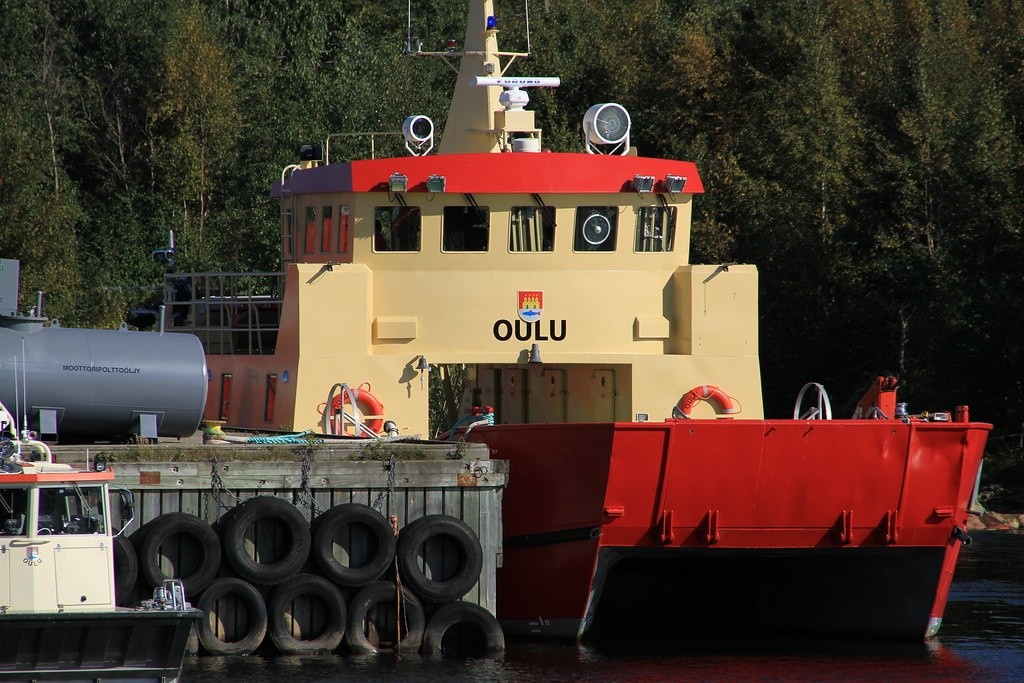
[123,1,998,656]
[1,428,203,683]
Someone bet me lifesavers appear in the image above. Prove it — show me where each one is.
[680,386,736,420]
[325,389,384,441]
[144,495,507,657]
[112,527,138,605]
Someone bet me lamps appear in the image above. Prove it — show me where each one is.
[666,174,688,201]
[425,173,446,193]
[93,460,106,471]
[388,173,408,193]
[0,441,15,459]
[298,144,323,161]
[633,174,656,198]
[582,102,632,156]
